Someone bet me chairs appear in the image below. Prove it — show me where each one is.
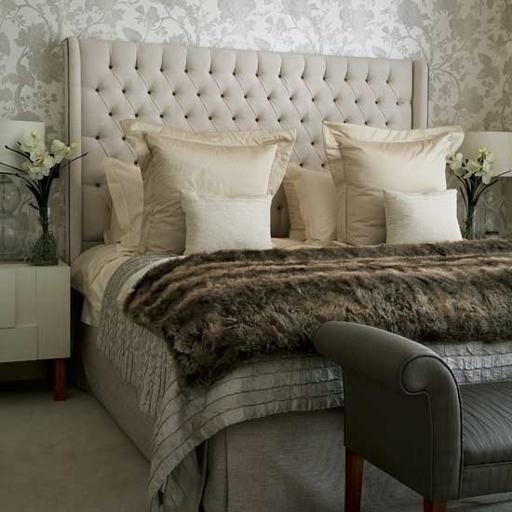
[313,319,512,512]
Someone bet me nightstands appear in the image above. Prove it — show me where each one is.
[1,257,71,400]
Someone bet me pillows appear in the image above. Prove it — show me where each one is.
[100,156,142,253]
[176,186,273,256]
[282,164,340,241]
[120,118,297,256]
[320,118,462,246]
[382,187,464,243]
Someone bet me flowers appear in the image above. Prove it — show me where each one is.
[0,129,90,266]
[447,146,511,241]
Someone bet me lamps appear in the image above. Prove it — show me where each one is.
[464,131,511,241]
[1,120,47,263]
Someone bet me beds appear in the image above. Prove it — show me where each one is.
[62,35,512,511]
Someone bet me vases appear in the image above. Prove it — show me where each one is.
[460,191,486,243]
[23,205,60,265]
